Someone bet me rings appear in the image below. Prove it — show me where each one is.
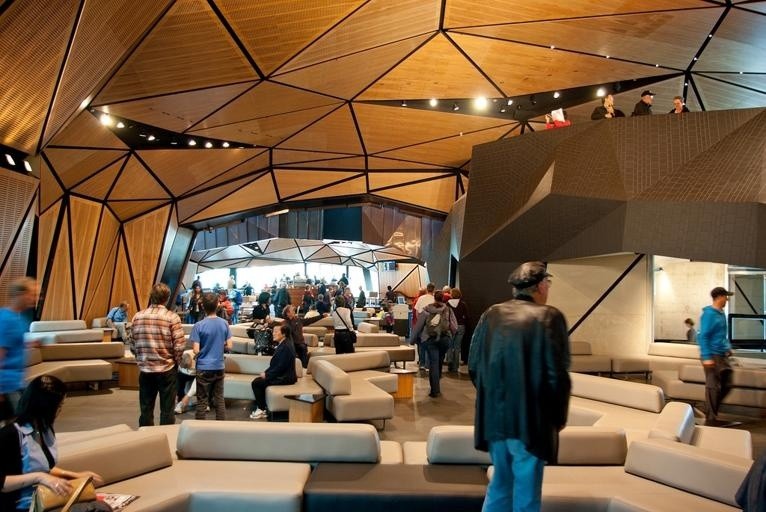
[54,483,59,489]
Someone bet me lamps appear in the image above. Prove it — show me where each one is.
[401,87,606,113]
[19,160,32,175]
[87,112,230,149]
[0,154,15,169]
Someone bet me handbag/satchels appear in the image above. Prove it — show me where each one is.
[253,326,273,354]
[350,331,357,343]
[34,477,97,512]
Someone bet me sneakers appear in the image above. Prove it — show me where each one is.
[249,408,268,419]
[174,401,188,414]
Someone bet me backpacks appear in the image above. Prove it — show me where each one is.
[426,313,447,336]
[233,289,243,305]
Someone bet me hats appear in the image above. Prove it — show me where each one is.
[507,260,553,290]
[641,90,656,97]
[711,287,734,298]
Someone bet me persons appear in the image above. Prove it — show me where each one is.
[697,286,735,427]
[130,284,186,428]
[0,277,38,423]
[0,375,112,511]
[247,271,467,375]
[172,279,243,421]
[684,318,697,343]
[409,291,458,398]
[544,110,572,130]
[630,90,654,118]
[467,262,571,512]
[590,93,626,120]
[105,302,130,345]
[249,324,297,419]
[667,96,690,114]
